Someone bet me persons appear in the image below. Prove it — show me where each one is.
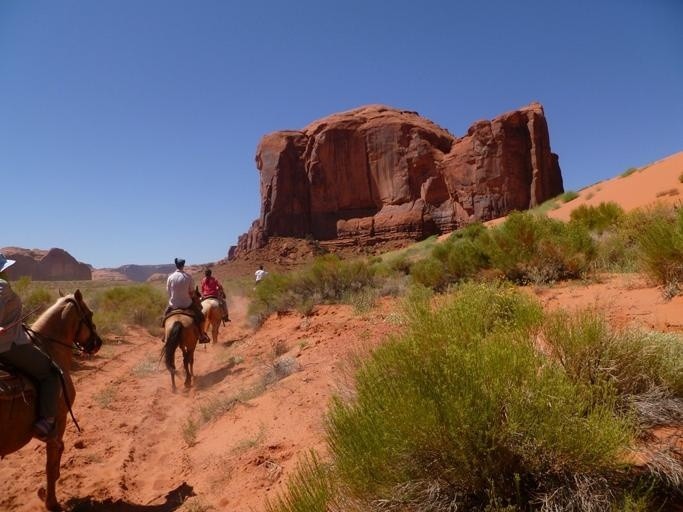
[1,254,64,441]
[200,269,231,322]
[164,259,210,344]
[255,266,268,282]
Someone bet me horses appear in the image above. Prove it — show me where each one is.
[0,289,102,512]
[159,285,201,393]
[201,298,224,352]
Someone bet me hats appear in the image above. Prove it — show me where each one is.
[0,254,17,272]
[175,257,185,266]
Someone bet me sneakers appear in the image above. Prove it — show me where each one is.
[35,418,61,449]
